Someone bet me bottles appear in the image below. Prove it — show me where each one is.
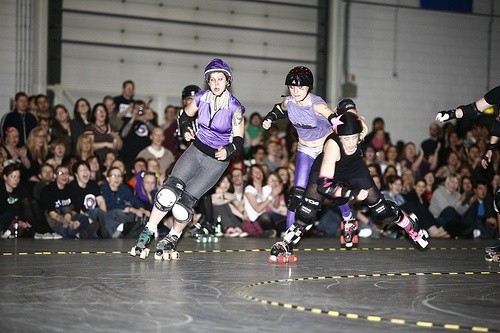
[12,216,19,238]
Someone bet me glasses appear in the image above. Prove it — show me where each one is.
[108,174,122,178]
[56,171,68,175]
[35,135,46,138]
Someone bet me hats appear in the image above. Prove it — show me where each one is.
[182,85,202,100]
[337,98,356,116]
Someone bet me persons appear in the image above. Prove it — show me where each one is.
[262,66,358,264]
[130,58,246,261]
[270,98,429,263]
[435,85,500,262]
[0,80,500,243]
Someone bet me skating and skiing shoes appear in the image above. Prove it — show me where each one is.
[129,226,156,260]
[154,231,182,261]
[186,216,220,244]
[406,212,430,251]
[268,240,299,265]
[340,218,358,250]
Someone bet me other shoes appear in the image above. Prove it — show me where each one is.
[224,226,249,238]
[264,228,277,239]
[466,228,482,239]
[33,232,63,240]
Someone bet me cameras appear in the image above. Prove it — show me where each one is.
[137,107,144,115]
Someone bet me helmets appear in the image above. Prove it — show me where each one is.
[285,66,314,90]
[203,57,232,87]
[332,112,363,136]
